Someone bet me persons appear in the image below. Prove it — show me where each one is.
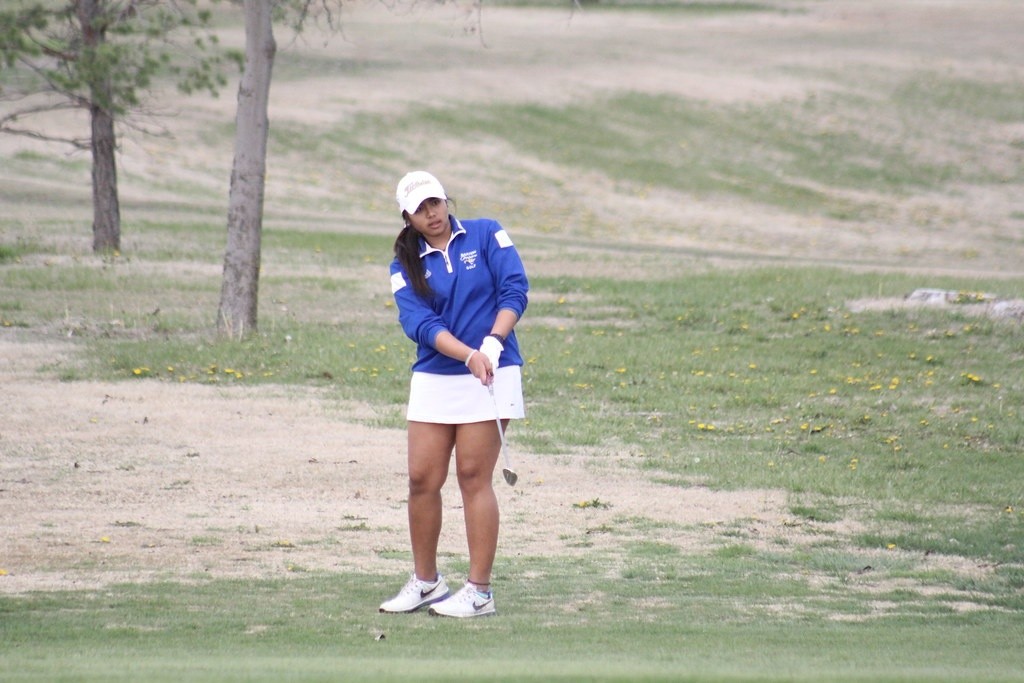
[378,170,528,619]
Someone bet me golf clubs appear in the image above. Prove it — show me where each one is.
[484,376,519,486]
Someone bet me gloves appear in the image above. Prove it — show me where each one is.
[479,335,504,375]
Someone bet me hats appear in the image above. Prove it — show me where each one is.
[396,171,447,214]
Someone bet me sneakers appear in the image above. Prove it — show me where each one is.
[379,573,449,614]
[429,581,496,617]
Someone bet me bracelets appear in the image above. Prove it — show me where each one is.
[465,349,478,367]
[490,334,504,346]
